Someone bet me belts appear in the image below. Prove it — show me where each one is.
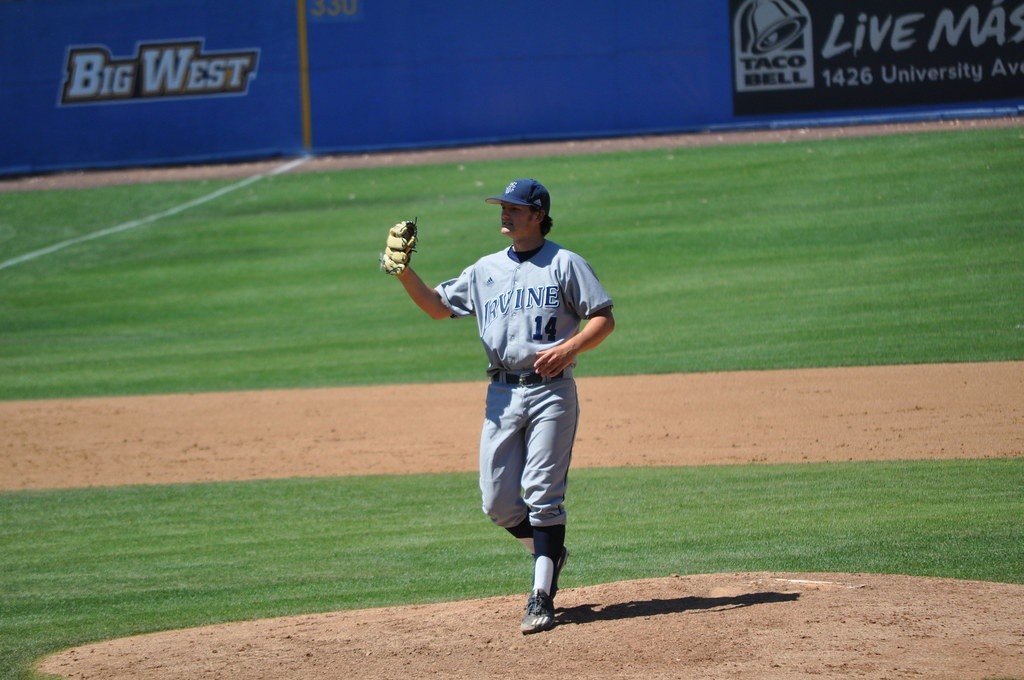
[492,369,563,385]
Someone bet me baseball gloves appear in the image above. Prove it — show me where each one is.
[381,215,418,276]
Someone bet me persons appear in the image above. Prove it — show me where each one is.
[380,178,617,634]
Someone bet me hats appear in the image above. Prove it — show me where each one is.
[485,179,550,211]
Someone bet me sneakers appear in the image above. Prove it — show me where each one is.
[520,588,555,633]
[532,546,569,589]
